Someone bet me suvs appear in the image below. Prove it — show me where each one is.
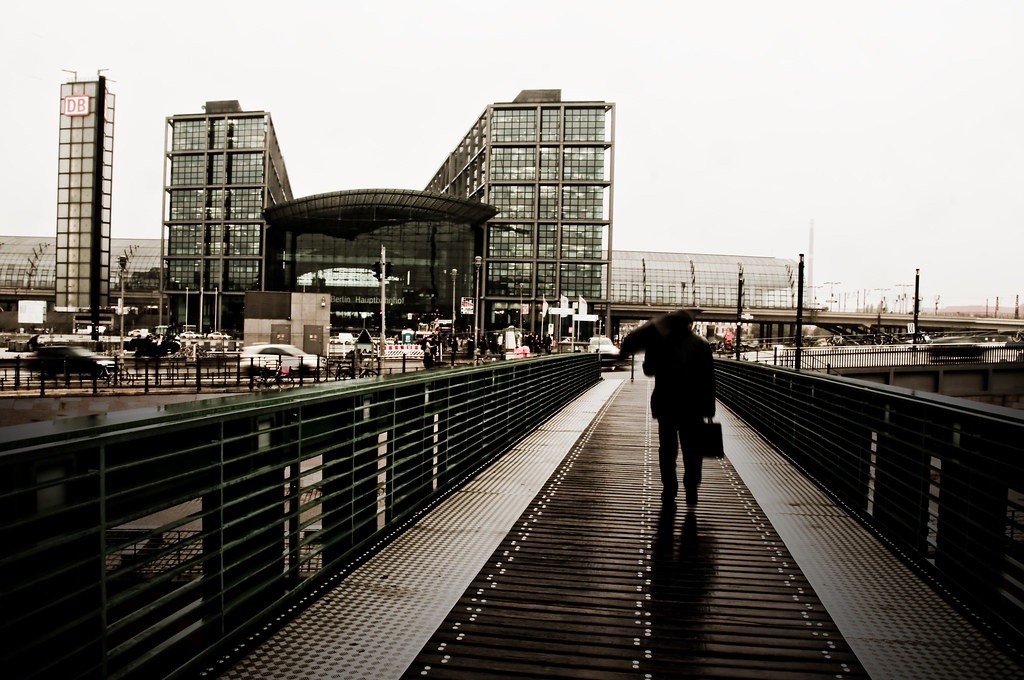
[239,344,327,371]
[24,345,124,377]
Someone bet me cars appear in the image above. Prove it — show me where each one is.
[330,336,348,344]
[180,331,204,339]
[128,329,142,337]
[206,331,233,340]
[588,337,620,355]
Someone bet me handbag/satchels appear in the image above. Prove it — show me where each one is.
[700,416,724,460]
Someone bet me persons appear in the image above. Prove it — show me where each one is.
[545,333,552,355]
[467,335,497,357]
[873,331,894,345]
[643,311,716,507]
[450,337,458,352]
[29,335,39,347]
[726,328,732,343]
[394,335,399,345]
[527,332,542,354]
[423,334,437,364]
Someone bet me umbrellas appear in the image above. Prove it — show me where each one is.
[618,309,704,363]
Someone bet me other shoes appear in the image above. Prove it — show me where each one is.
[684,481,697,506]
[661,489,677,501]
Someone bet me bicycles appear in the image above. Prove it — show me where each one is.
[82,354,131,386]
[314,358,379,382]
[254,360,294,390]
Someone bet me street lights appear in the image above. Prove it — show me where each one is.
[474,256,482,350]
[449,268,458,340]
[519,283,524,334]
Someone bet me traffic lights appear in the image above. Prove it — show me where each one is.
[371,262,380,282]
[386,262,395,279]
